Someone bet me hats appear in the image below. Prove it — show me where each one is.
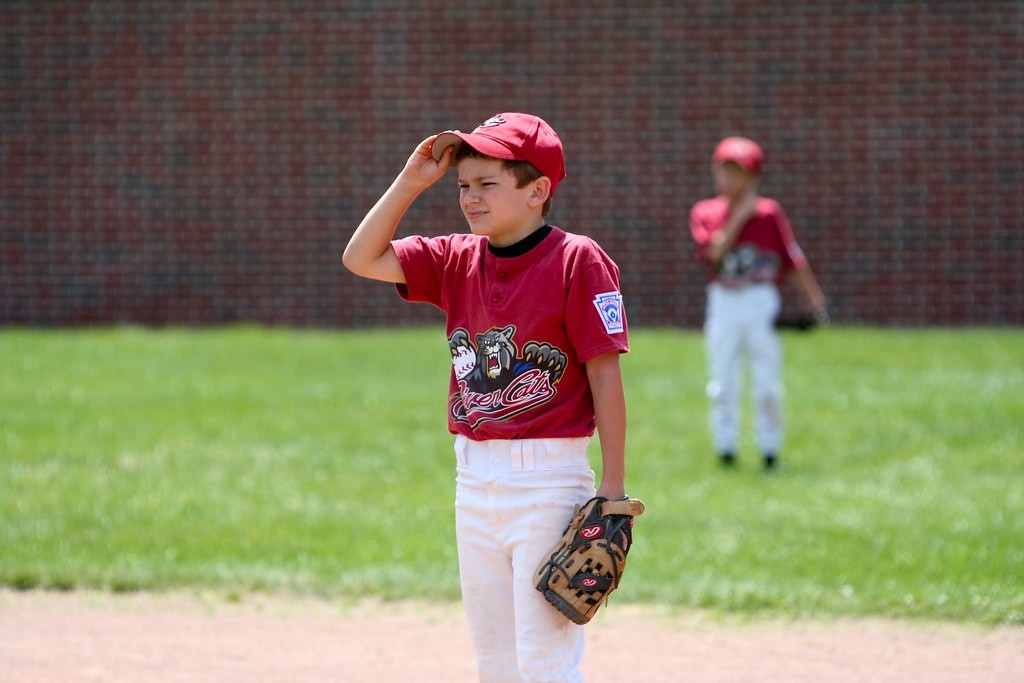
[431,112,567,198]
[714,137,763,174]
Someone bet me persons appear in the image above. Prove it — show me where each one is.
[342,110,644,682]
[688,135,830,470]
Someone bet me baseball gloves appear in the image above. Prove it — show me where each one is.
[774,308,818,331]
[530,493,646,626]
